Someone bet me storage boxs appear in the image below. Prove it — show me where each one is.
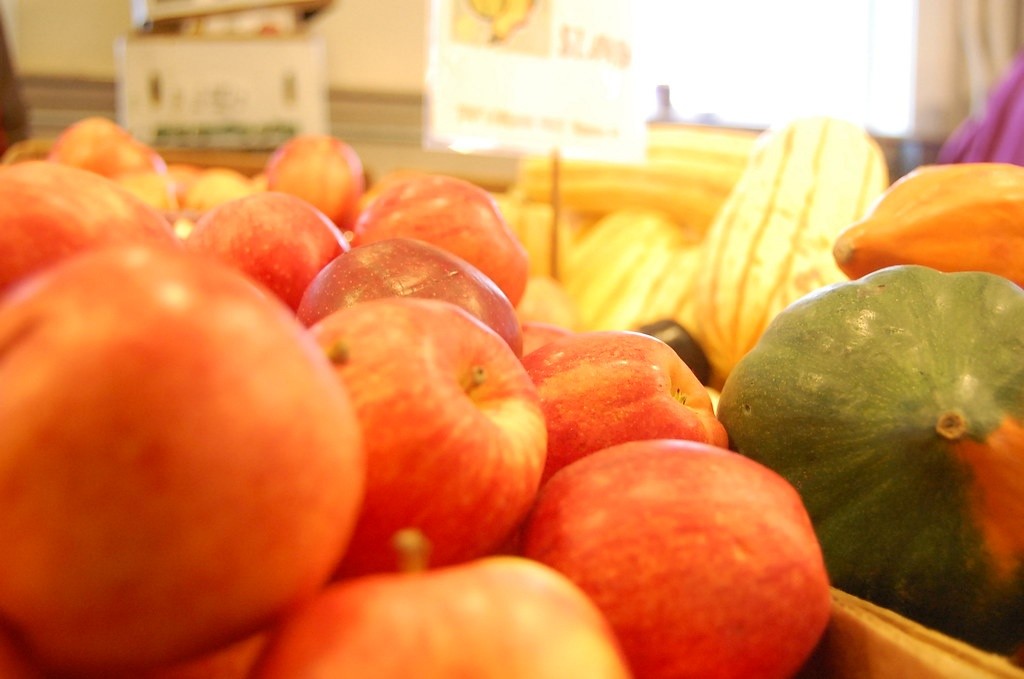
[114,0,332,150]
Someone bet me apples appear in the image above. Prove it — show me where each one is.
[0,118,833,679]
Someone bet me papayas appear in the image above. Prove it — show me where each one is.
[481,106,1024,662]
